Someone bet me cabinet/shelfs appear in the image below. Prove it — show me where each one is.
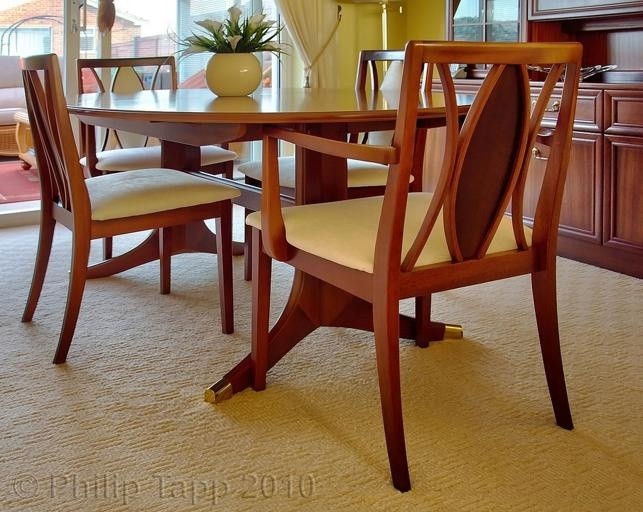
[446,0,643,279]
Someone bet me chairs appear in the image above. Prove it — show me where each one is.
[237,47,438,282]
[73,55,240,264]
[244,41,584,494]
[18,52,243,365]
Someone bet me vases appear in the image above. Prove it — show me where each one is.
[204,51,264,97]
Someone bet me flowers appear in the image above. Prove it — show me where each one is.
[165,3,297,56]
